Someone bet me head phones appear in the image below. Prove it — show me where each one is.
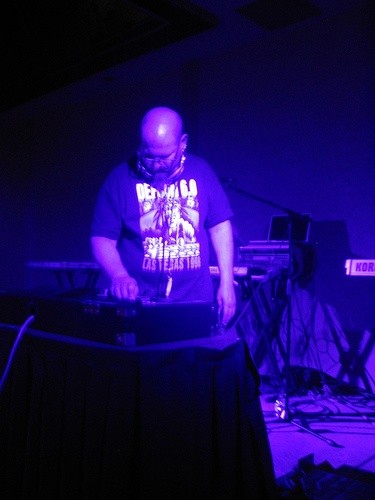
[137,154,186,182]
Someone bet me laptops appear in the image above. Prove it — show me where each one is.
[248,213,312,244]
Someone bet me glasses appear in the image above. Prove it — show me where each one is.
[142,142,180,165]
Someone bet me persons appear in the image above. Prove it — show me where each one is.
[86,107,236,326]
